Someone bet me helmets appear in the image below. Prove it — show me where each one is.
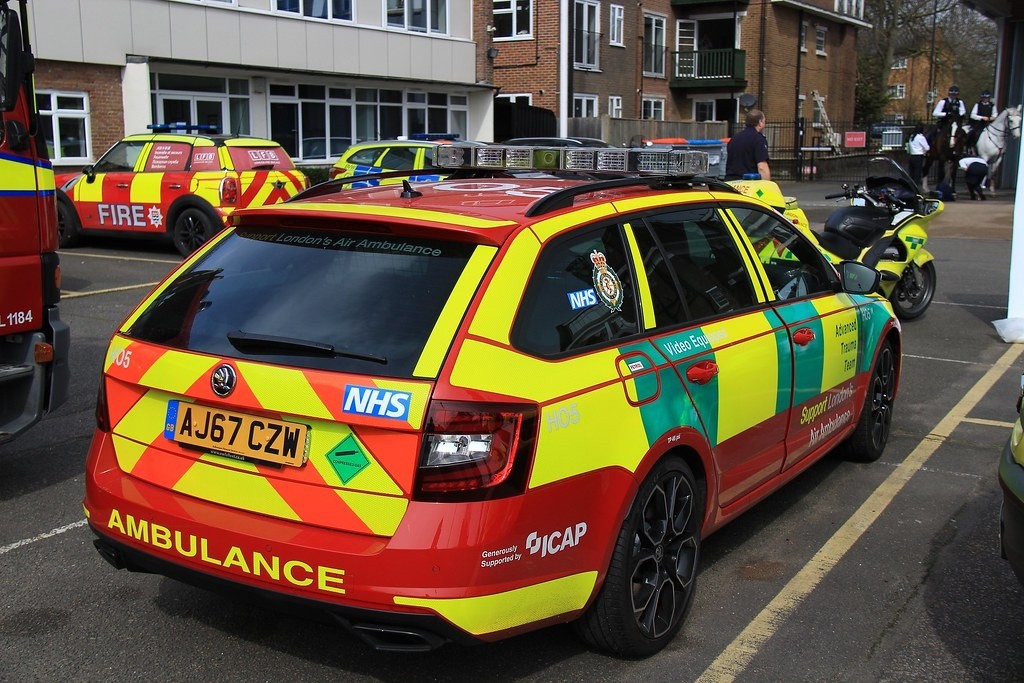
[948,86,959,95]
[980,90,992,98]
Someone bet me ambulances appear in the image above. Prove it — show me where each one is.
[328,132,514,191]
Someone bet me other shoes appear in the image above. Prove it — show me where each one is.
[971,196,977,200]
[981,197,986,200]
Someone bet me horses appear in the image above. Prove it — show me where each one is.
[922,103,1023,201]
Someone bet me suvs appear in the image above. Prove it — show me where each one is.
[52,121,314,259]
[78,143,906,659]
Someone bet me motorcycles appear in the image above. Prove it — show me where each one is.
[721,156,946,323]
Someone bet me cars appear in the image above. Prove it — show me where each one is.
[302,136,365,160]
[502,136,639,180]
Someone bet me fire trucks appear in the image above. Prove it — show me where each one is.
[0,0,74,445]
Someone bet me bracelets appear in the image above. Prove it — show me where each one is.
[988,116,990,122]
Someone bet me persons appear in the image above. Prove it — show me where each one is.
[723,108,772,183]
[907,85,1001,200]
